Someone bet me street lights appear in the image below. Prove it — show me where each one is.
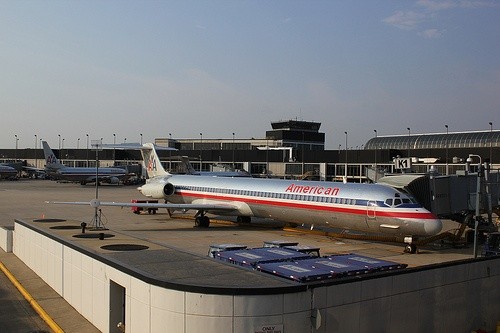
[231,132,236,169]
[407,128,411,157]
[374,129,377,184]
[199,133,203,171]
[488,122,493,169]
[13,134,143,169]
[344,131,348,164]
[168,133,172,170]
[444,124,449,177]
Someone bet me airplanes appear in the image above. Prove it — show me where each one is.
[0,141,136,186]
[42,143,443,254]
